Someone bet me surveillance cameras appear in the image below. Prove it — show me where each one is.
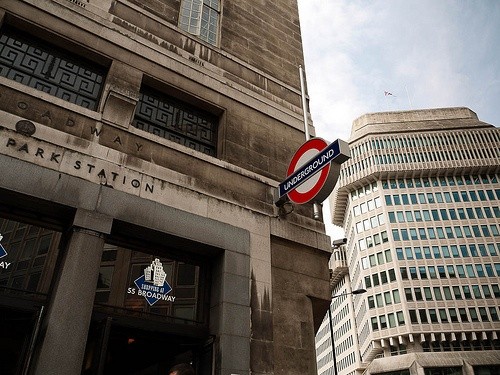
[332,238,347,251]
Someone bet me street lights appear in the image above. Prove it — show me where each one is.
[328,289,368,375]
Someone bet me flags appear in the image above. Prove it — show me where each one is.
[385,91,392,96]
